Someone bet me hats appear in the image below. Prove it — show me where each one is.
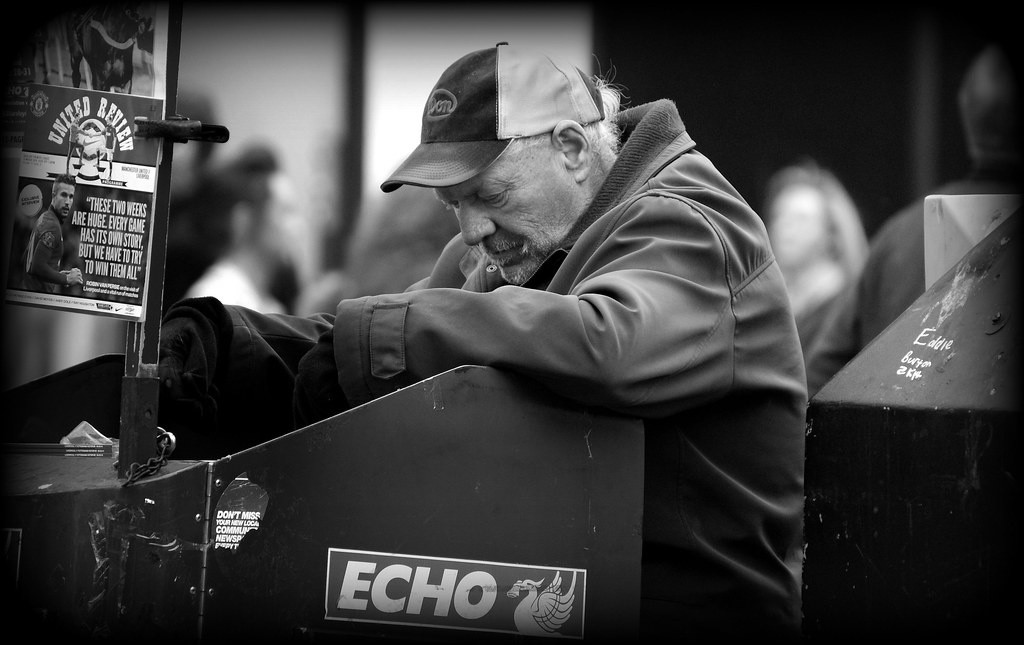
[379,42,608,192]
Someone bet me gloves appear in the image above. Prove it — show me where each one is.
[288,327,351,428]
[152,307,222,428]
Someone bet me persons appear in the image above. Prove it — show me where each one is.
[64,110,117,181]
[157,41,875,645]
[21,174,85,292]
